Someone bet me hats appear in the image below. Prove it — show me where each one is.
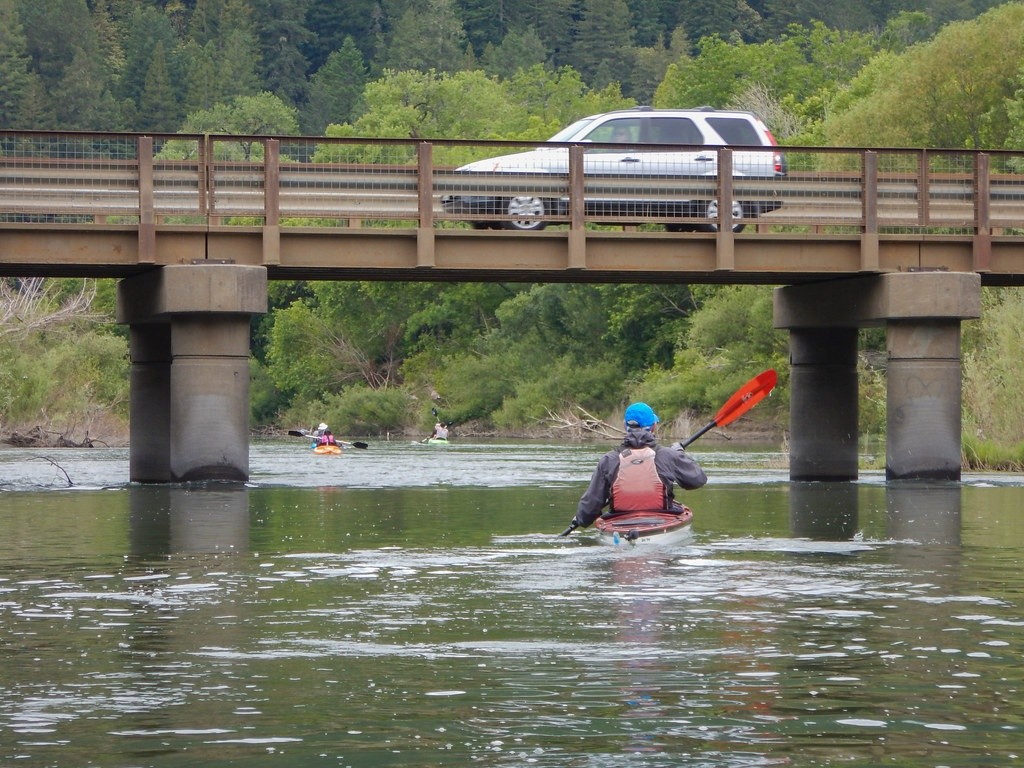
[625,402,660,428]
[435,423,440,429]
[318,423,328,430]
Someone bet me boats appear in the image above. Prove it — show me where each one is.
[428,438,450,445]
[594,502,697,547]
[314,445,342,455]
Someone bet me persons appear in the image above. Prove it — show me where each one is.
[427,423,449,440]
[313,423,336,445]
[571,404,707,528]
[609,125,633,142]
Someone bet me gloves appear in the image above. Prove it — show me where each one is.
[670,442,684,453]
[572,515,578,526]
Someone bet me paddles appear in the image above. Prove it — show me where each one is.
[552,371,779,536]
[431,407,440,422]
[286,429,368,449]
[420,421,460,446]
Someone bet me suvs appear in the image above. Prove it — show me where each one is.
[440,105,788,230]
